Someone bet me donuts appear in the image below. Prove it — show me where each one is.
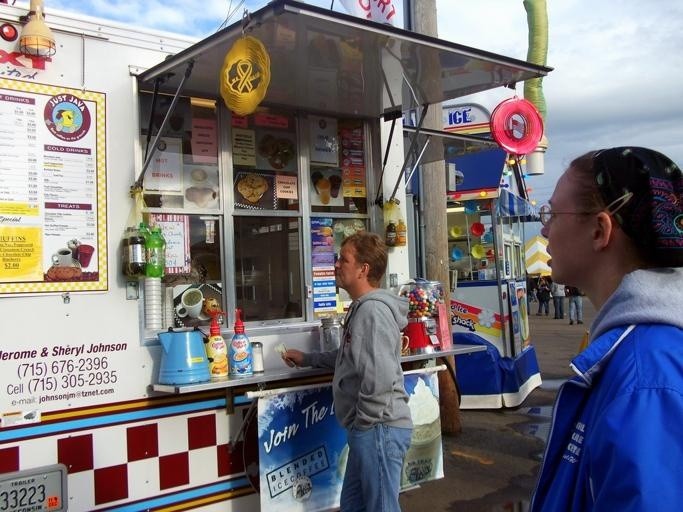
[269,138,294,169]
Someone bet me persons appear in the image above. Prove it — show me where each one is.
[283,232,413,512]
[526,274,585,324]
[530,146,683,512]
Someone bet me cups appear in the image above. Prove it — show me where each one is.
[67,239,95,268]
[51,248,72,268]
[311,170,342,205]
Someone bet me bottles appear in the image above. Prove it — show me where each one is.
[250,341,264,373]
[318,317,343,354]
[121,221,166,279]
[385,218,407,246]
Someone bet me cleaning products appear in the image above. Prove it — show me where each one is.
[206,307,229,377]
[229,308,253,376]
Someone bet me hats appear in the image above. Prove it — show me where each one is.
[592,147,683,267]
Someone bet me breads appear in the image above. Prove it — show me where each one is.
[237,174,268,203]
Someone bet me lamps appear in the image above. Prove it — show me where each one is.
[20,0,56,58]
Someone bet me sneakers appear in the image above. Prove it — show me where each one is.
[536,312,564,319]
[569,320,583,325]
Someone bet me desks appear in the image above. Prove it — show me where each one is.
[150,344,488,414]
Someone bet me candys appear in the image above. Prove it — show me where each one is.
[400,287,445,318]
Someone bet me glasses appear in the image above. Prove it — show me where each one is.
[538,205,596,226]
